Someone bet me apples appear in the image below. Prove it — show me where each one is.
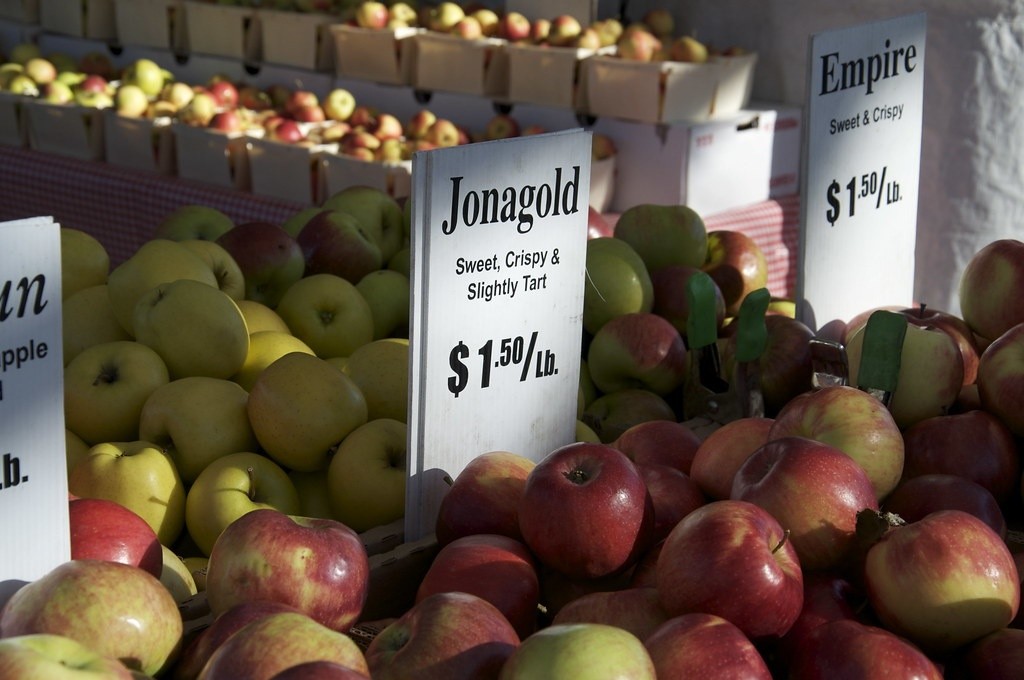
[0,39,615,169]
[0,183,1024,680]
[276,0,710,64]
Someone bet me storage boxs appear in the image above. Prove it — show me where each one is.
[415,31,510,95]
[588,51,761,125]
[106,109,169,172]
[0,0,39,23]
[184,1,261,62]
[246,135,322,207]
[330,22,414,87]
[26,101,107,162]
[396,149,615,220]
[169,125,247,184]
[40,0,119,38]
[115,0,188,49]
[317,152,389,210]
[256,9,335,71]
[503,42,581,109]
[0,92,43,147]
